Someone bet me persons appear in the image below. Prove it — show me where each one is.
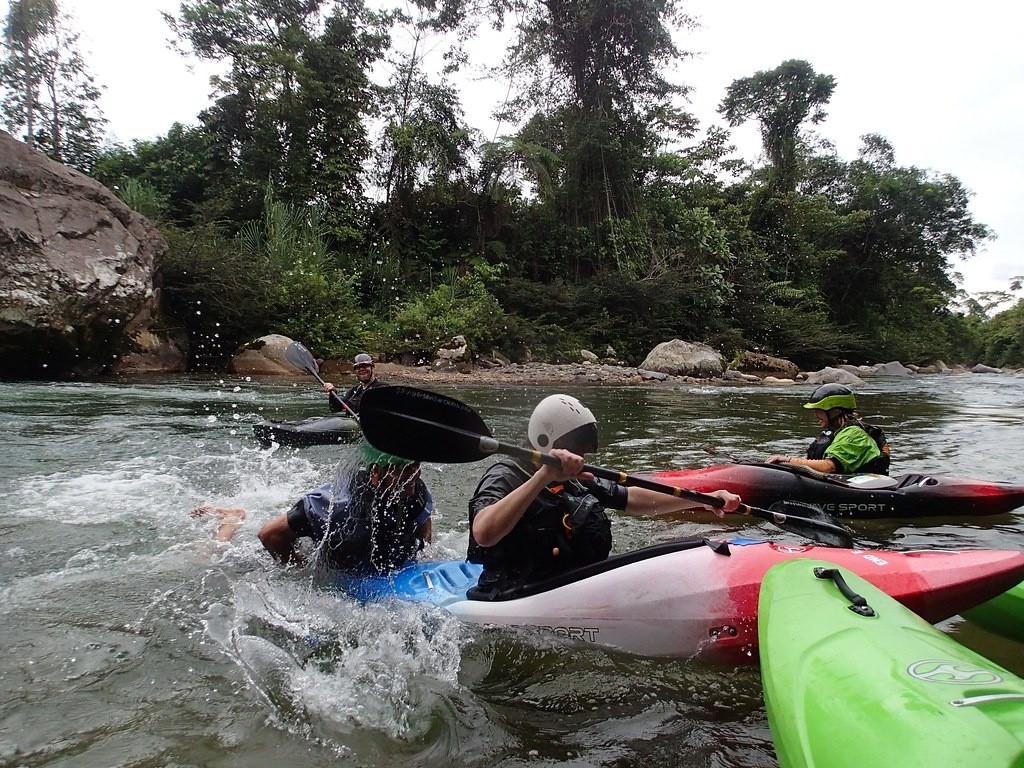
[764,383,890,480]
[323,354,392,418]
[187,435,438,577]
[463,394,742,595]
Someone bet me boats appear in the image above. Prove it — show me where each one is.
[254,413,365,450]
[316,534,1024,664]
[956,581,1023,643]
[756,554,1024,768]
[611,456,1024,529]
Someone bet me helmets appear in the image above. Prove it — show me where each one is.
[363,441,416,476]
[353,355,374,369]
[802,382,857,412]
[527,393,598,457]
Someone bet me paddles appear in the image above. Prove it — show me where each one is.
[358,385,855,549]
[779,462,899,490]
[287,341,360,425]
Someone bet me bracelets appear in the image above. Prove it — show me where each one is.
[787,457,792,462]
